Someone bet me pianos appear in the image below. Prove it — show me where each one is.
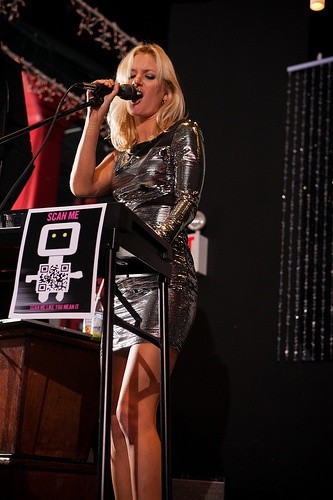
[1,203,173,277]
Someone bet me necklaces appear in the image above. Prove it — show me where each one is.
[111,127,166,173]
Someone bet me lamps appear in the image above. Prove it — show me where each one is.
[309,0,326,11]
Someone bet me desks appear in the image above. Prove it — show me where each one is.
[0,318,114,499]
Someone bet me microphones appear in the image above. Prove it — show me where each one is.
[74,80,138,100]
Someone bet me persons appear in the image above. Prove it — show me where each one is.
[69,43,206,500]
